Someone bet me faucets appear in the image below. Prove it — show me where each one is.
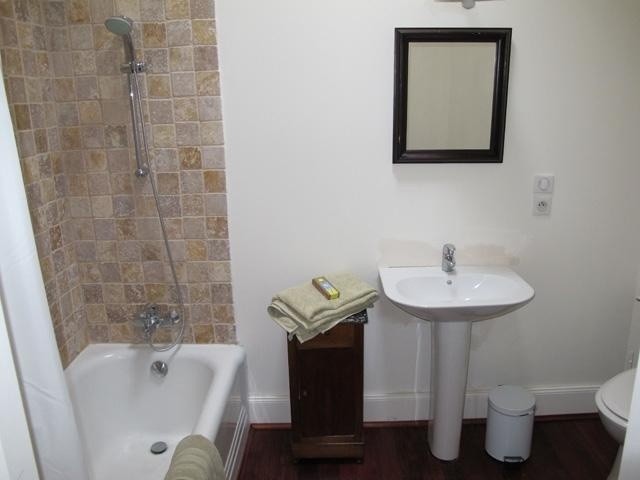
[441,244,457,272]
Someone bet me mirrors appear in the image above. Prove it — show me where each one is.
[392,25,514,163]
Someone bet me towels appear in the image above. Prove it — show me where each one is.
[269,273,377,342]
[166,435,226,479]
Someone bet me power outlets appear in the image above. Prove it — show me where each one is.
[533,196,552,216]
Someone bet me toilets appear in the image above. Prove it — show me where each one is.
[593,366,635,480]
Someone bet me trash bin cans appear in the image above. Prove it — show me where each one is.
[484,385,535,463]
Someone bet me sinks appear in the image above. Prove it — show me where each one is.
[378,265,535,321]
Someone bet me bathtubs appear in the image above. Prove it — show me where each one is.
[64,342,250,480]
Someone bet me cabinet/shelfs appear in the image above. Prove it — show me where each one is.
[287,309,368,459]
